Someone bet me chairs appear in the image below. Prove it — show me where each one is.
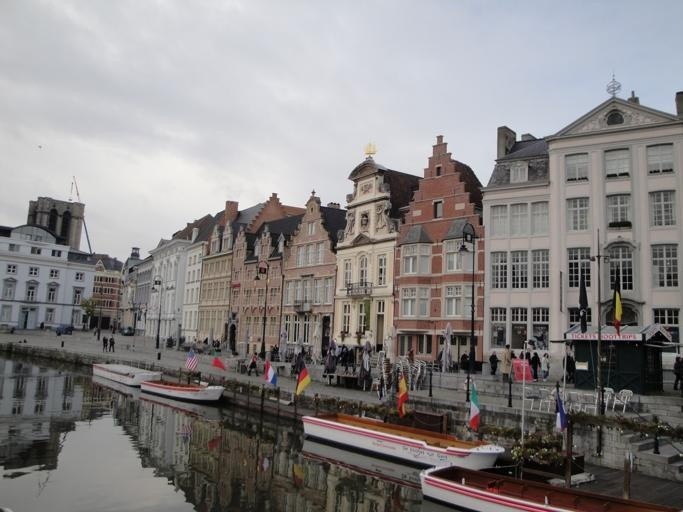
[370,351,427,400]
[525,388,634,415]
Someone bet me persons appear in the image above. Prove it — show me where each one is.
[344,213,355,237]
[108,334,116,352]
[437,348,444,370]
[248,351,261,377]
[269,344,275,361]
[489,343,551,383]
[274,343,279,359]
[562,350,576,383]
[102,335,109,352]
[406,346,416,363]
[672,356,682,390]
[376,206,387,229]
[460,349,468,370]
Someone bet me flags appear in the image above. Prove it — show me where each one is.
[396,370,410,420]
[390,494,405,512]
[296,366,312,396]
[264,356,277,385]
[254,447,270,474]
[292,462,305,487]
[185,347,200,372]
[212,356,225,370]
[612,262,623,337]
[554,384,568,434]
[207,437,223,449]
[577,262,590,334]
[176,421,193,437]
[469,379,480,432]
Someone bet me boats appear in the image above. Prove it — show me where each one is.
[140,380,225,401]
[302,413,506,471]
[92,363,161,387]
[419,466,670,511]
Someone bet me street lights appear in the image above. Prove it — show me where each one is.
[459,221,474,401]
[254,260,270,359]
[150,274,163,350]
